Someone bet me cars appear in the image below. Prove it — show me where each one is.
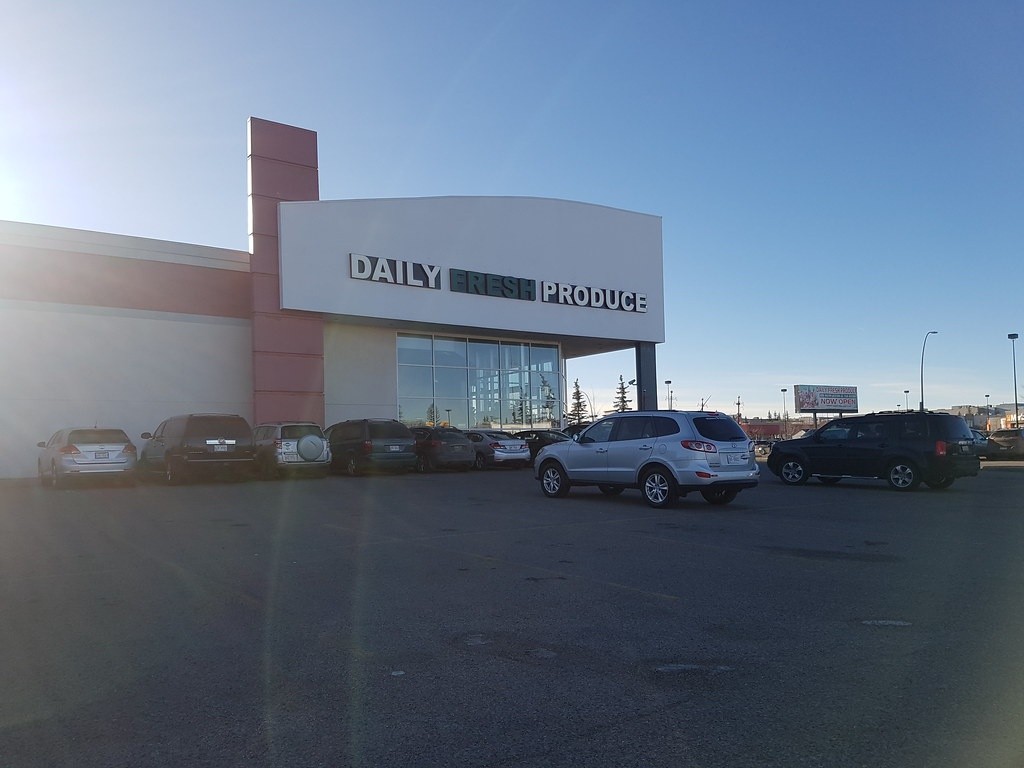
[751,440,774,457]
[36,425,139,490]
[561,423,606,441]
[970,428,988,457]
[509,429,572,468]
[462,429,531,471]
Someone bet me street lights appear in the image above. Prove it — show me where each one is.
[734,401,741,425]
[904,390,910,412]
[984,395,990,430]
[1007,332,1019,428]
[445,409,453,427]
[665,380,672,410]
[780,389,787,441]
[921,331,939,411]
[897,405,901,412]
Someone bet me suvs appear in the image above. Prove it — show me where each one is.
[321,416,420,477]
[407,425,477,474]
[532,409,761,509]
[767,410,979,493]
[986,426,1024,460]
[255,420,334,479]
[138,411,258,484]
